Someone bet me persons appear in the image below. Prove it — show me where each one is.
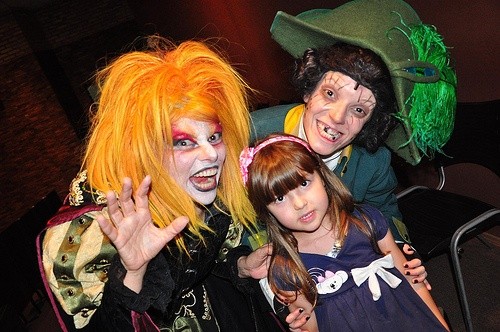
[31,32,290,330]
[240,131,453,332]
[232,41,431,291]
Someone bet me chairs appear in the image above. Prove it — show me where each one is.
[389,157,500,332]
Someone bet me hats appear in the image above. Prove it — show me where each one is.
[269,2,456,166]
